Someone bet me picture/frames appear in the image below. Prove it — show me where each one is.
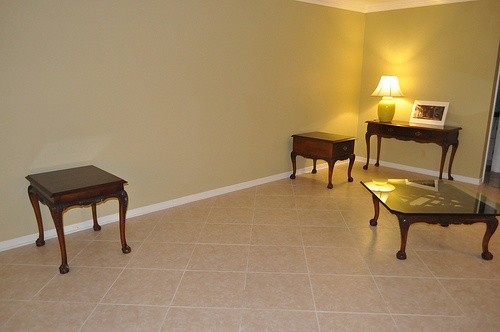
[410,100,450,126]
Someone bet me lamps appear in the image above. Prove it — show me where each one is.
[371,76,404,122]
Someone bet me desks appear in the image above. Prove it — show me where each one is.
[360,178,500,259]
[25,165,131,273]
[365,120,462,180]
[290,130,356,189]
